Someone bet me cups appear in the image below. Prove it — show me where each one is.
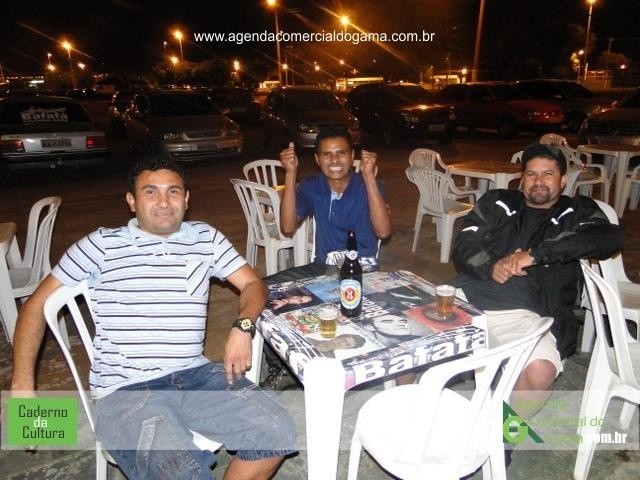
[436,283,456,316]
[316,303,339,337]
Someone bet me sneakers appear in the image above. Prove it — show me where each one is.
[258,365,301,400]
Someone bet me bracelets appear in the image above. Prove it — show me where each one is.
[528,247,536,266]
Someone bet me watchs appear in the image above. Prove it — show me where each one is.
[231,317,256,340]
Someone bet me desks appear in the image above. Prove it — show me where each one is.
[0,222,22,346]
[581,144,640,216]
[244,263,488,480]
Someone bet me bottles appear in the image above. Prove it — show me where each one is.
[340,230,363,318]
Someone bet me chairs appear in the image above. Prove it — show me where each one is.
[580,197,640,354]
[573,257,640,479]
[42,279,225,480]
[229,133,610,276]
[618,165,640,219]
[8,196,71,351]
[347,316,554,480]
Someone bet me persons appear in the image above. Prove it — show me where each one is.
[304,334,365,361]
[9,156,298,480]
[361,311,421,351]
[393,143,622,473]
[262,294,312,313]
[258,126,391,392]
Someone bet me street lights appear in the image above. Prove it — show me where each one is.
[163,41,167,58]
[45,41,86,91]
[174,30,184,64]
[284,56,349,88]
[268,0,283,82]
[232,58,242,86]
[605,34,615,72]
[342,15,348,80]
[575,47,584,81]
[583,0,597,79]
